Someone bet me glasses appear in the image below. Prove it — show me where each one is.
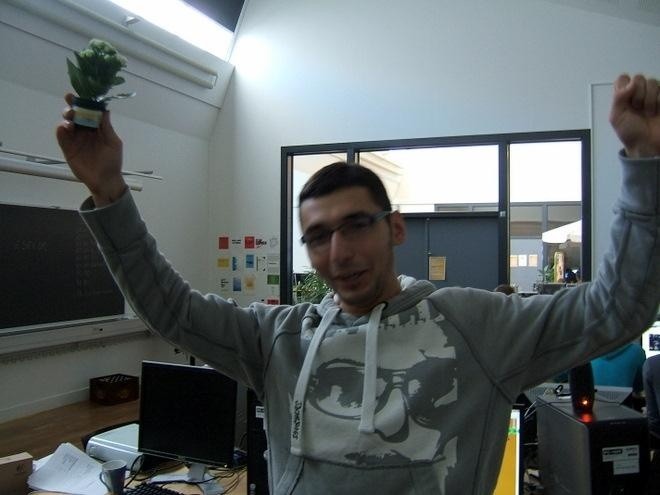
[300,211,391,251]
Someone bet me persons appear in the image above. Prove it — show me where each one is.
[643,353,660,465]
[590,334,646,412]
[56,75,660,495]
[493,285,514,295]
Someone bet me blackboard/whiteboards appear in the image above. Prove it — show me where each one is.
[0,201,124,334]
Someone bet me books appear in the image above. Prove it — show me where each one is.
[27,441,114,495]
[87,424,170,469]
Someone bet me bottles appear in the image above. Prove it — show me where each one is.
[569,359,595,414]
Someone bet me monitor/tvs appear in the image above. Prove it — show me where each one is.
[136,359,237,495]
[490,404,525,495]
[641,319,660,360]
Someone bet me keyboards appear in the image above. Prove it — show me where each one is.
[121,482,186,495]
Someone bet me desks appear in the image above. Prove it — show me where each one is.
[0,381,248,495]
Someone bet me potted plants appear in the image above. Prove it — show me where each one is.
[64,37,136,133]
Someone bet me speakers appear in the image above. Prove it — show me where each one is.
[568,363,596,416]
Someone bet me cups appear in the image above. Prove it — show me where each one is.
[99,460,127,495]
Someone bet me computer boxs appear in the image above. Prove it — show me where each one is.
[536,392,649,495]
[247,386,270,495]
